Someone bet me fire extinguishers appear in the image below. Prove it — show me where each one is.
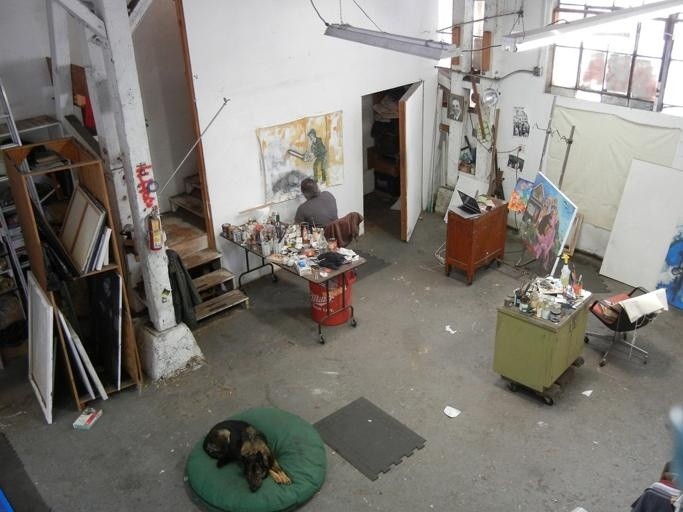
[145,206,163,250]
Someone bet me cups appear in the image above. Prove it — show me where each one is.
[572,281,582,296]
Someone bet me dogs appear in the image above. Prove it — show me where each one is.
[202,420,292,493]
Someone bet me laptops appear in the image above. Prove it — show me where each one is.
[451,190,482,219]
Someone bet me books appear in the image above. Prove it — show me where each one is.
[72,407,103,431]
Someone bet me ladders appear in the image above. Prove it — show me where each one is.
[0,81,47,320]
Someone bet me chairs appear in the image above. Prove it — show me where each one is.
[584,285,669,367]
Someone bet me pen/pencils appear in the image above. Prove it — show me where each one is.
[570,264,582,286]
[518,281,531,294]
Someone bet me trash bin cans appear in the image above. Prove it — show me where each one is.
[309,270,358,327]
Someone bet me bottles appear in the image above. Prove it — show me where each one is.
[327,238,337,252]
[515,289,561,324]
[300,222,310,248]
[221,211,281,258]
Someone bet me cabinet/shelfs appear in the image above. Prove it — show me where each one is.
[491,276,592,394]
[444,194,509,286]
[0,135,144,410]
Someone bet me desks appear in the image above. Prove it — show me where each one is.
[218,217,369,344]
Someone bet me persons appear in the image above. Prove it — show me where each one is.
[96,273,115,321]
[307,128,330,185]
[517,178,529,200]
[524,197,560,269]
[462,152,472,163]
[449,97,462,121]
[293,178,340,228]
